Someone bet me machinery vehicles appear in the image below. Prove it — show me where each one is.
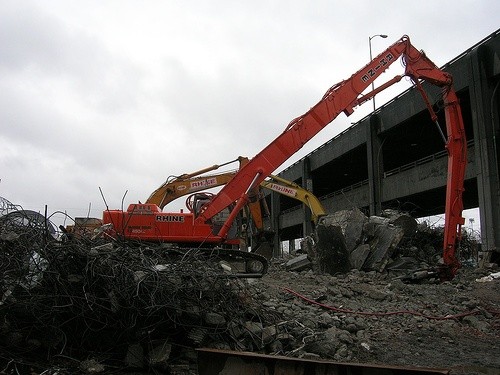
[104,33,468,285]
[64,156,328,261]
